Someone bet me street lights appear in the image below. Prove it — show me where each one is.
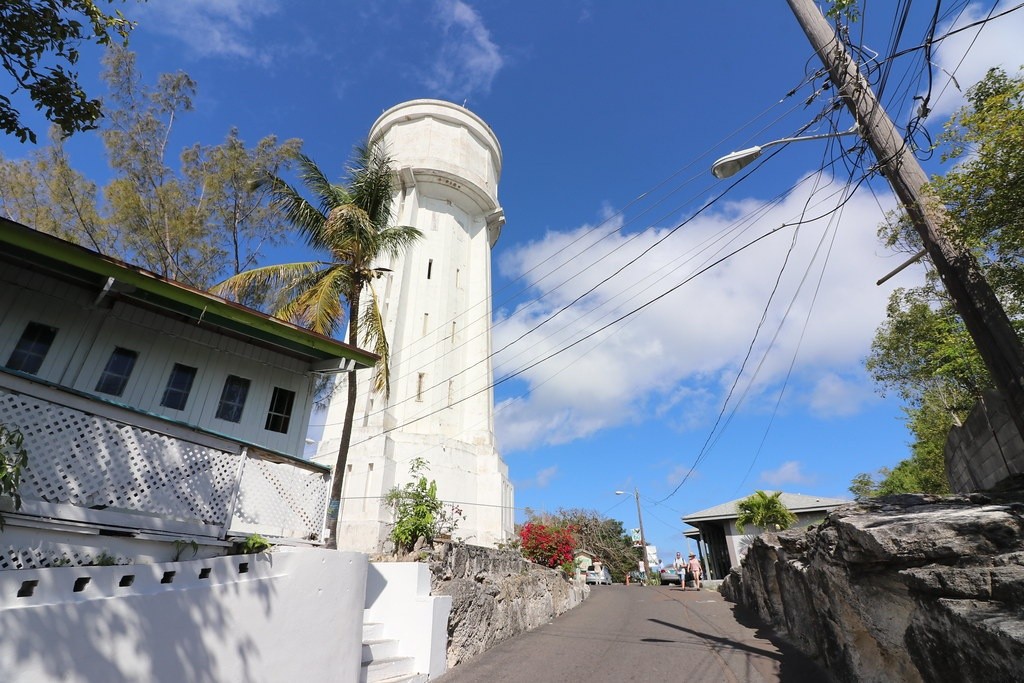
[614,487,652,586]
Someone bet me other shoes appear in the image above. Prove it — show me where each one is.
[697,588,700,591]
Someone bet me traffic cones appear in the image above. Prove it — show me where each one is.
[625,575,629,586]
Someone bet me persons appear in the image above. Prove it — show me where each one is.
[636,558,645,584]
[673,552,688,590]
[687,552,704,591]
[654,558,663,584]
[592,556,602,583]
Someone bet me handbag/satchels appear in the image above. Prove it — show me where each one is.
[699,570,702,580]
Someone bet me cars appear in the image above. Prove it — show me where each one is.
[586,565,612,586]
[661,564,685,586]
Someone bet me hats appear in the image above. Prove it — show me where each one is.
[688,552,696,557]
